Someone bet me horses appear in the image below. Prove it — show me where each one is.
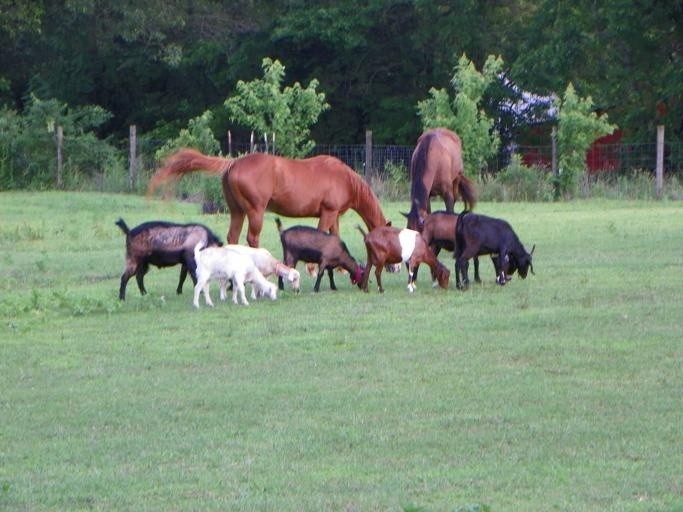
[145,148,402,280]
[398,127,478,233]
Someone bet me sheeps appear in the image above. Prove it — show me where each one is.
[219,244,301,304]
[411,197,518,284]
[114,218,224,302]
[192,240,278,308]
[273,217,372,294]
[453,210,536,291]
[354,224,451,293]
